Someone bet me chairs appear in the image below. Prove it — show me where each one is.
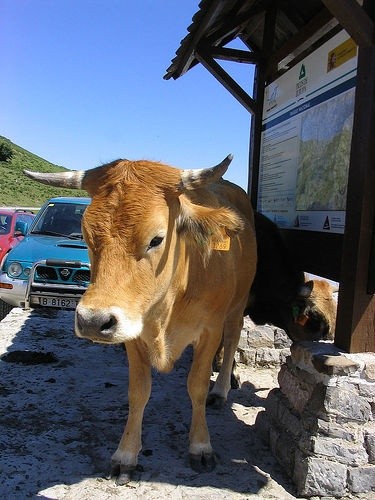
[57,215,81,239]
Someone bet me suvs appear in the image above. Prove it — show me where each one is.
[1,198,92,309]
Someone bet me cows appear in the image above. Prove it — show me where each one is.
[286,280,337,343]
[22,154,259,486]
[213,209,331,390]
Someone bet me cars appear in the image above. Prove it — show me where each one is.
[0,208,36,263]
